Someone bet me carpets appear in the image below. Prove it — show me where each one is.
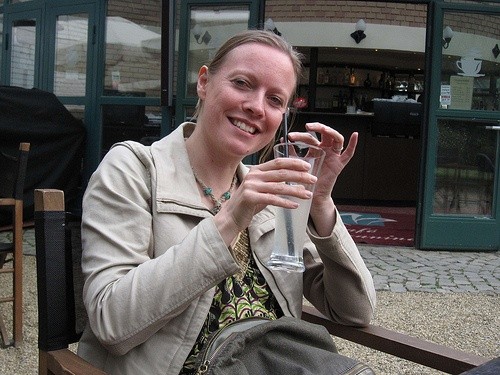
[338,210,416,246]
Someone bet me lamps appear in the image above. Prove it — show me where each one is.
[350,19,367,43]
[443,27,453,49]
[265,17,281,35]
[192,24,211,45]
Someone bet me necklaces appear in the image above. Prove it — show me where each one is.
[190,167,237,213]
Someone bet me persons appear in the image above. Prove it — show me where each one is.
[81,29,377,375]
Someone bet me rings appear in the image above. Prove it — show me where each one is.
[331,147,343,151]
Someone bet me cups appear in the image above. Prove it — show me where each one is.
[267,142,327,273]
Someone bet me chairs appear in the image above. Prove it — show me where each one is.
[35,189,489,375]
[0,143,30,346]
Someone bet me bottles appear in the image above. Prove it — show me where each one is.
[364,73,371,87]
[379,74,384,88]
[316,91,368,111]
[317,65,361,86]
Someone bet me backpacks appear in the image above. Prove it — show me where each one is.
[195,315,375,375]
